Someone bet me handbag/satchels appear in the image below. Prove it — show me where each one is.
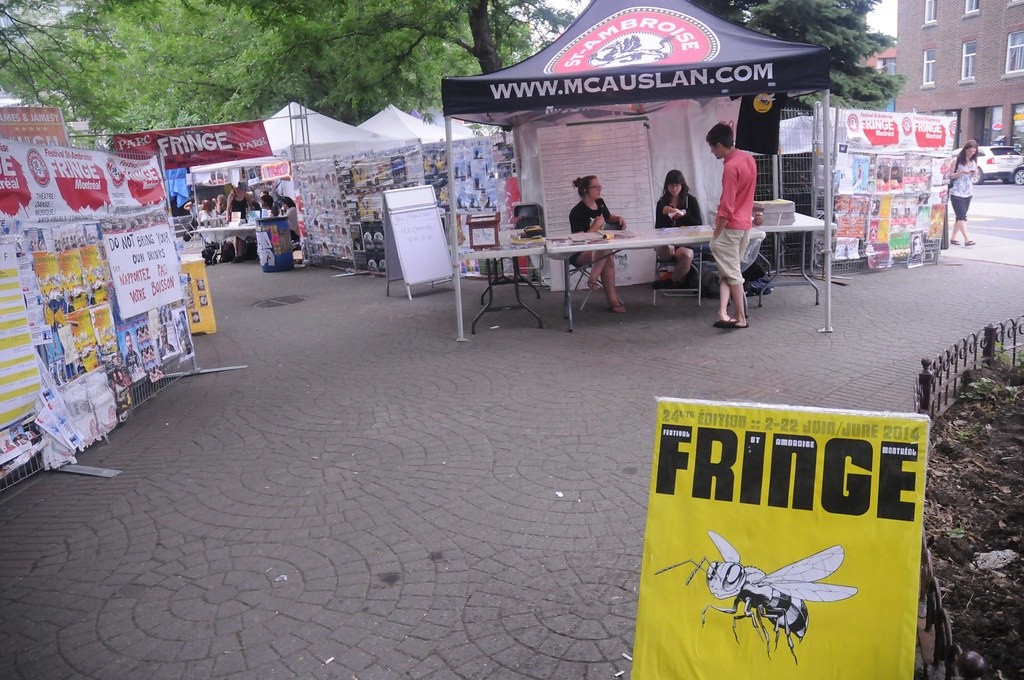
[949,156,959,188]
[220,242,235,263]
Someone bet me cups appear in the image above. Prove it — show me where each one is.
[508,230,521,241]
[204,217,226,228]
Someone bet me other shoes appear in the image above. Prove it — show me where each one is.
[652,279,676,289]
[690,264,699,274]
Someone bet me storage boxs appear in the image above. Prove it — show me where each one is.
[466,211,501,249]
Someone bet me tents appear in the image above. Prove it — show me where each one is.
[441,0,835,342]
[356,103,476,148]
[190,99,422,249]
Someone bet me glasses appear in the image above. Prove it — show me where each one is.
[588,185,602,189]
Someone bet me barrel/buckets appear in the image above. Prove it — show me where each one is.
[255,216,294,273]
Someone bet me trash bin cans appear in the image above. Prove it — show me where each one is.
[255,217,295,273]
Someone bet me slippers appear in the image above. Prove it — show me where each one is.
[965,240,976,245]
[587,280,599,290]
[610,304,626,313]
[951,240,961,245]
[714,317,749,328]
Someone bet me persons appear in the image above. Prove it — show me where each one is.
[226,181,253,263]
[183,194,227,263]
[246,190,262,210]
[124,331,141,371]
[911,234,924,261]
[569,176,627,314]
[280,197,299,244]
[949,140,979,248]
[261,190,274,210]
[652,169,703,289]
[706,122,757,328]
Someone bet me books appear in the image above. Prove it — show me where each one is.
[569,228,636,245]
[750,197,796,225]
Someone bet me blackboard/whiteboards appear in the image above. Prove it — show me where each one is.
[384,184,454,286]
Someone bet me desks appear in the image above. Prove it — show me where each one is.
[181,258,217,335]
[545,211,838,334]
[461,228,545,335]
[189,224,256,267]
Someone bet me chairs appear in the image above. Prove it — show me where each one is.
[569,261,624,312]
[651,245,703,306]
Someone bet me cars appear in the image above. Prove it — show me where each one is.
[1010,164,1024,186]
[952,145,1024,185]
